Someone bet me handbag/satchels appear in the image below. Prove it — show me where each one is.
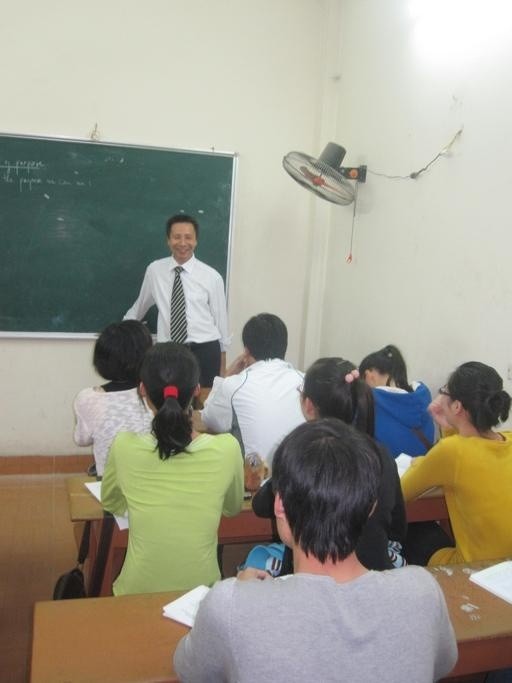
[52,568,87,600]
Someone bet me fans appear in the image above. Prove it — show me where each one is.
[281,138,371,207]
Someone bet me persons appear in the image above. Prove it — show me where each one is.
[252,359,407,575]
[99,342,246,597]
[358,345,436,461]
[198,313,308,478]
[72,322,155,483]
[122,214,234,388]
[172,420,458,682]
[399,362,512,563]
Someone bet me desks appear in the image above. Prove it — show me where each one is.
[69,468,449,597]
[31,559,512,683]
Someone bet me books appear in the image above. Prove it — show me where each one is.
[468,560,512,604]
[394,452,442,500]
[161,584,210,633]
[85,478,130,534]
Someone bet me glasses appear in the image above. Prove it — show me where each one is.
[439,384,458,397]
[296,385,306,396]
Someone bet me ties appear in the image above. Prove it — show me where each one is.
[170,266,188,345]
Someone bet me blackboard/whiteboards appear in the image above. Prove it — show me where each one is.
[0,132,238,340]
